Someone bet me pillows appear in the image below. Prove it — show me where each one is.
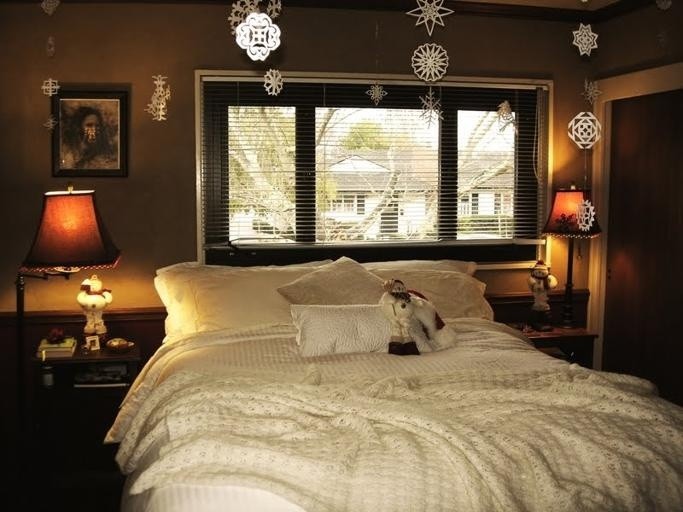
[360,259,478,279]
[277,257,391,305]
[149,266,334,345]
[288,302,399,345]
[156,259,334,273]
[366,268,495,325]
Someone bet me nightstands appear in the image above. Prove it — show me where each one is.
[28,343,144,509]
[518,325,598,372]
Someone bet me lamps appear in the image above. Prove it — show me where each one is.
[6,188,118,510]
[545,189,601,328]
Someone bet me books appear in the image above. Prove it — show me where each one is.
[36,336,78,359]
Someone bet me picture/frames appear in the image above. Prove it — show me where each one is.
[52,81,130,180]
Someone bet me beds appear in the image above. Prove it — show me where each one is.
[100,255,682,510]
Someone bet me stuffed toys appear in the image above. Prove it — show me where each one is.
[527,260,559,312]
[379,278,457,353]
[76,275,113,335]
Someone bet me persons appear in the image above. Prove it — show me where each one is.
[61,106,118,169]
